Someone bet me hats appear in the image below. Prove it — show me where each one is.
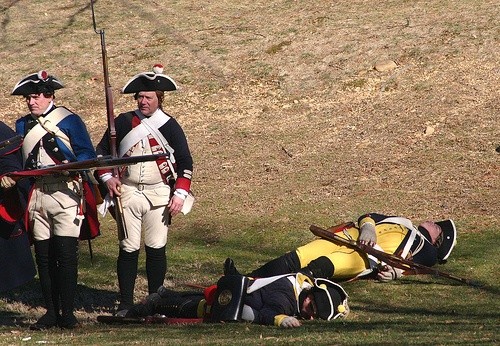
[435,219,456,260]
[314,278,349,322]
[123,72,178,93]
[10,70,65,95]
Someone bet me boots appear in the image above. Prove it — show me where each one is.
[118,249,141,309]
[30,238,61,329]
[292,256,334,280]
[224,250,301,277]
[145,245,166,294]
[136,286,204,318]
[53,235,79,328]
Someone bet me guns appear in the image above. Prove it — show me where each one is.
[12,152,171,181]
[91,0,128,241]
[309,224,500,295]
[0,135,23,150]
